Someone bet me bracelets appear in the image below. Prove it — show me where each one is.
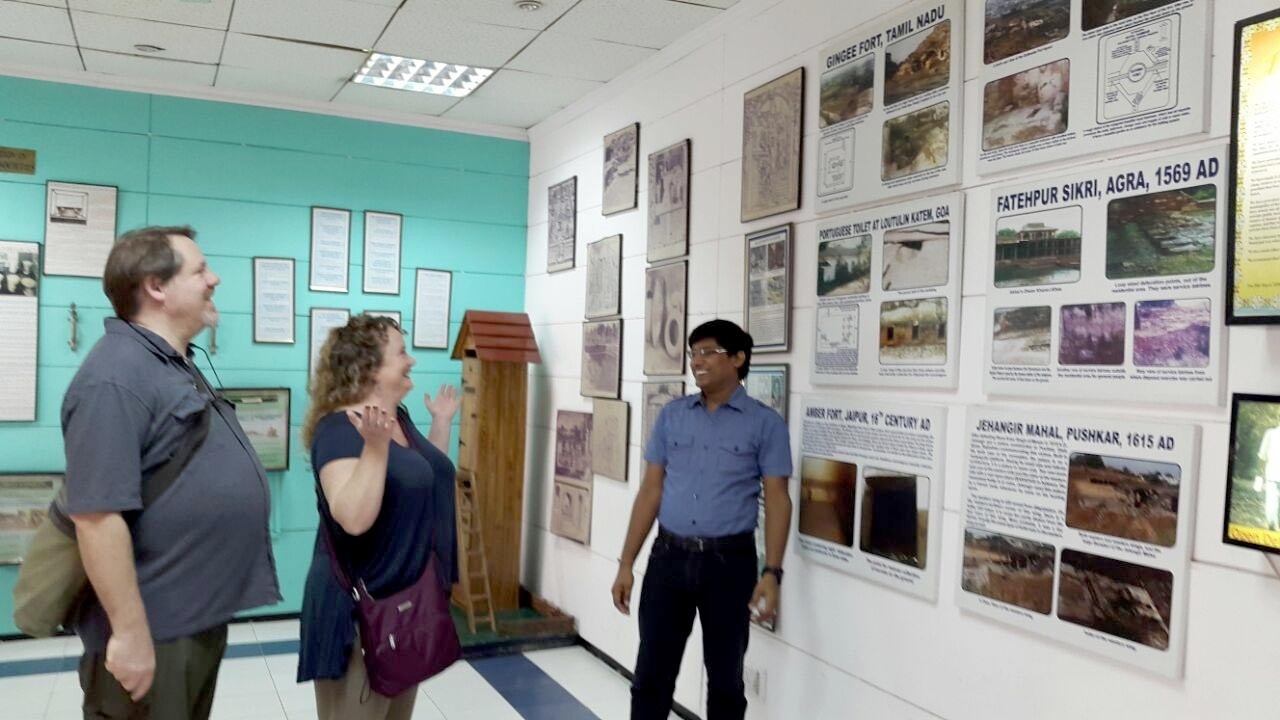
[617,558,633,566]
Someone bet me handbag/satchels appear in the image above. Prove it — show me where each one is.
[12,484,88,640]
[356,554,463,698]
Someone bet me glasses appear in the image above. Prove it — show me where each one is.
[686,348,728,360]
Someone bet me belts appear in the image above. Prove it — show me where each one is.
[657,524,755,552]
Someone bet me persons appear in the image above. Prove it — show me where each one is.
[294,313,462,719]
[1255,415,1280,528]
[62,228,282,720]
[611,320,794,720]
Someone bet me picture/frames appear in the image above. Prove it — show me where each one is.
[1221,392,1280,554]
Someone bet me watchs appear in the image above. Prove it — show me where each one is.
[761,566,783,584]
